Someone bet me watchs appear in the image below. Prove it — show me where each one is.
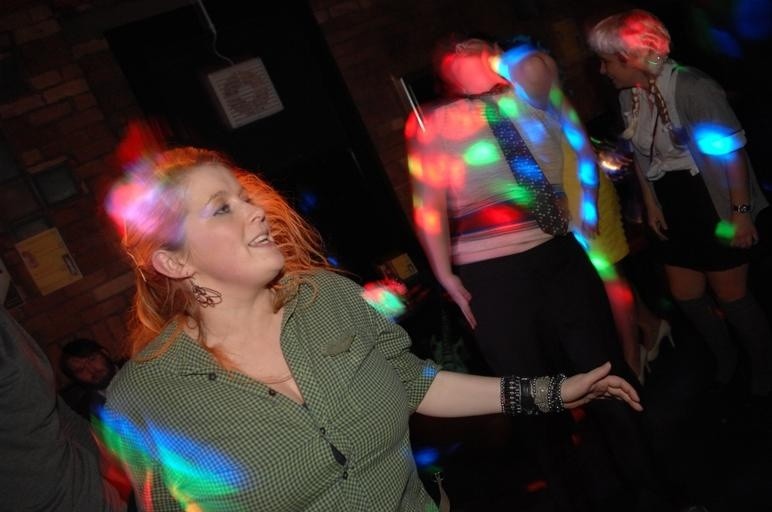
[731,203,751,214]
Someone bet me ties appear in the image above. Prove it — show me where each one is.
[480,94,568,238]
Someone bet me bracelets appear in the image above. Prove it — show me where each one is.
[498,371,568,416]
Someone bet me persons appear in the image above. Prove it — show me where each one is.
[403,36,674,510]
[48,337,131,420]
[0,300,137,511]
[583,9,772,399]
[95,144,646,510]
[480,35,677,390]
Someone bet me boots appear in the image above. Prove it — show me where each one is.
[675,294,740,385]
[719,291,772,396]
